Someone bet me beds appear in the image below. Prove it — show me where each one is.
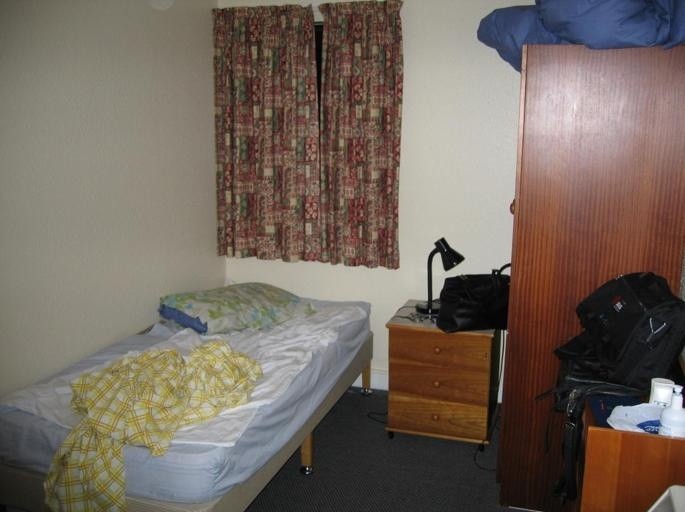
[1,281,374,512]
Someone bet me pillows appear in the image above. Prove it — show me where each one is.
[157,280,298,335]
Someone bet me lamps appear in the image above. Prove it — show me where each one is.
[415,237,465,317]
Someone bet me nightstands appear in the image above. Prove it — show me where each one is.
[385,298,497,447]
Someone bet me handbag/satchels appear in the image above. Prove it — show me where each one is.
[437,263,511,333]
[535,272,685,417]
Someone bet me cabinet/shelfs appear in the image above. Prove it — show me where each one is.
[496,39,685,512]
